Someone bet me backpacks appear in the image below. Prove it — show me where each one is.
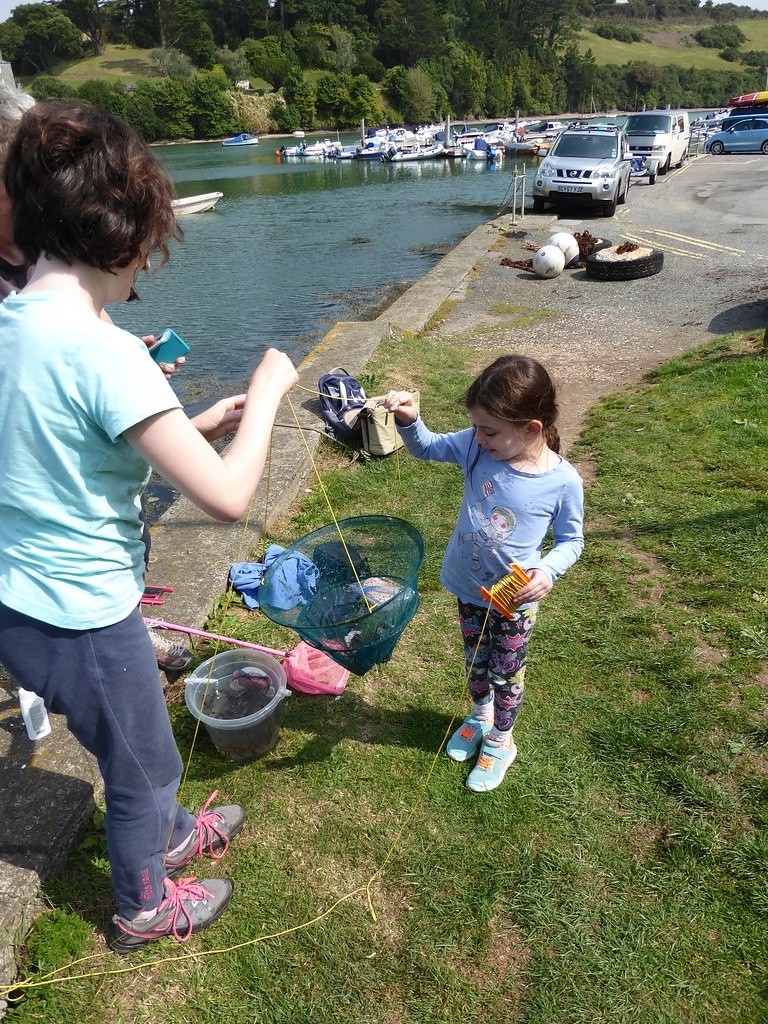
[318,366,367,451]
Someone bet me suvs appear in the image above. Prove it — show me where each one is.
[532,123,635,217]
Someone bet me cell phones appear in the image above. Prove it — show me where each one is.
[146,329,190,369]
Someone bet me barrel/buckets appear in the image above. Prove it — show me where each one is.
[184,649,292,763]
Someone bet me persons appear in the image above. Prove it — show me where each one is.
[1,97,300,952]
[0,85,197,675]
[383,355,583,793]
[513,127,524,143]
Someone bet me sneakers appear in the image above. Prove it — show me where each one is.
[143,617,196,673]
[108,877,234,955]
[465,734,517,792]
[164,791,245,881]
[446,712,493,762]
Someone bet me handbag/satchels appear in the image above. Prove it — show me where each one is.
[361,389,421,455]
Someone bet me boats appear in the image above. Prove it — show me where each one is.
[728,91,768,107]
[171,192,224,214]
[221,133,258,146]
[276,116,565,161]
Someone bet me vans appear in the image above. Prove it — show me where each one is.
[622,109,690,176]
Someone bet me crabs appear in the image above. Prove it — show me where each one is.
[376,623,394,638]
[202,667,279,761]
[344,630,363,654]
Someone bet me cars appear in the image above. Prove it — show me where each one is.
[705,118,768,155]
